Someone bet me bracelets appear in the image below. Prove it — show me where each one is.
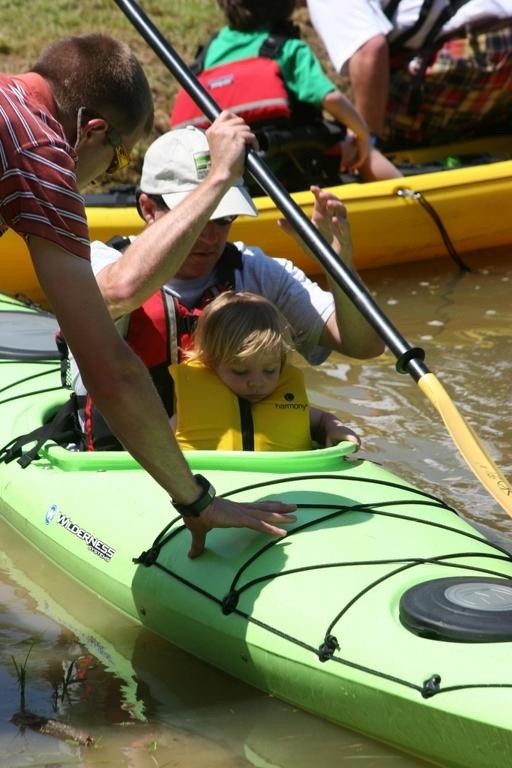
[171,473,217,517]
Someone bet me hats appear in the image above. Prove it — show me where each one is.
[140,124,258,219]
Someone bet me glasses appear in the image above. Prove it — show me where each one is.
[214,213,236,226]
[81,106,131,173]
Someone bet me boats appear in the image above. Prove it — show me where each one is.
[0,131,512,309]
[0,289,512,766]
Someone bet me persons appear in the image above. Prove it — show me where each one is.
[69,111,385,451]
[303,0,512,155]
[164,289,361,454]
[1,33,297,560]
[171,0,404,198]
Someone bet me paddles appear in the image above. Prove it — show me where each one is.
[119,1,512,516]
